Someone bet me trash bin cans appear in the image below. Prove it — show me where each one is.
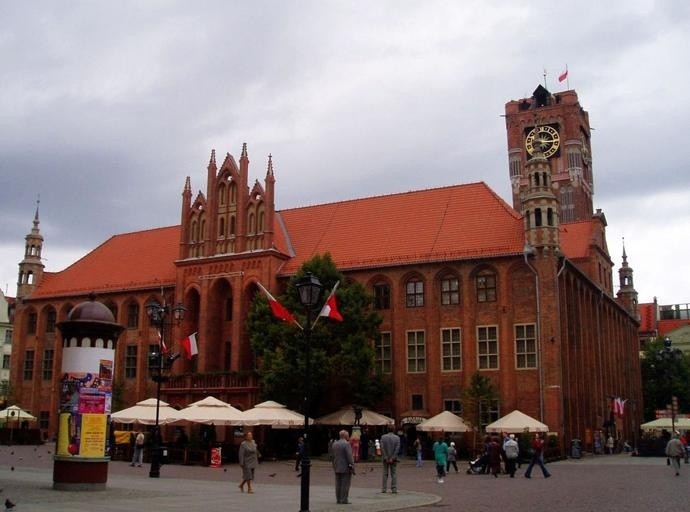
[570,439,582,459]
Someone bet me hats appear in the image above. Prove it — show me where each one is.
[450,442,455,447]
[509,433,515,439]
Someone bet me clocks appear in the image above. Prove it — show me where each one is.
[525,125,561,160]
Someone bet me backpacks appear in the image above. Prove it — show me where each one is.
[506,442,519,460]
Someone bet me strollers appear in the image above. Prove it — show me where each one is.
[467,452,487,475]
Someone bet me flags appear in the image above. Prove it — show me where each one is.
[558,71,568,83]
[178,333,199,360]
[264,291,294,322]
[319,291,343,322]
[156,331,168,353]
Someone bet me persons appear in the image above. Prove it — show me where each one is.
[330,430,354,504]
[129,428,146,467]
[413,438,424,469]
[594,429,689,477]
[294,436,304,471]
[445,441,458,473]
[432,437,449,485]
[379,424,401,493]
[470,431,553,480]
[238,431,261,494]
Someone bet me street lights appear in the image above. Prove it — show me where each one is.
[290,270,324,511]
[144,295,187,478]
[657,335,681,437]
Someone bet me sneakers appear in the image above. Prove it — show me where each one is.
[238,484,244,493]
[137,464,142,467]
[337,501,352,504]
[675,473,680,476]
[392,490,399,493]
[381,490,387,493]
[544,473,552,479]
[478,471,517,479]
[129,463,135,467]
[247,489,254,494]
[437,471,459,484]
[524,473,532,479]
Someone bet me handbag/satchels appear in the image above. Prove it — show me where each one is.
[666,457,671,466]
[136,444,144,449]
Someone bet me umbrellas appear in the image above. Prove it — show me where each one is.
[639,416,690,432]
[0,403,38,423]
[485,408,549,468]
[313,403,395,428]
[414,409,478,443]
[231,400,313,429]
[166,396,242,426]
[111,396,185,427]
